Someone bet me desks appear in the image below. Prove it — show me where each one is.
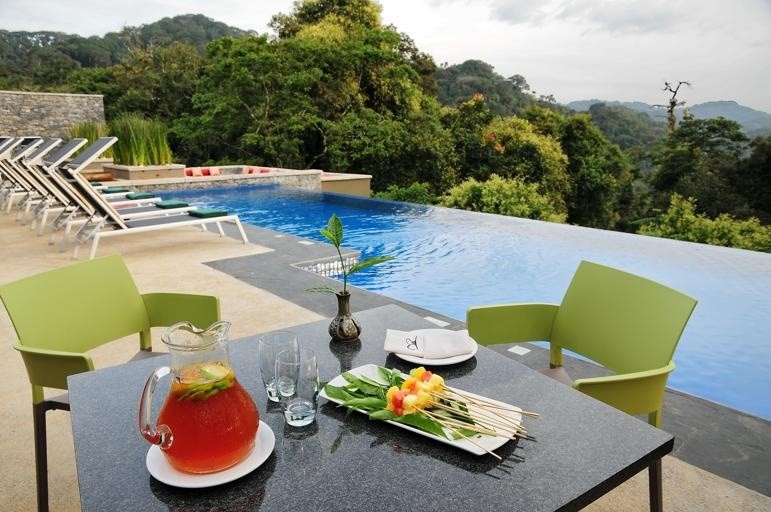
[67,304,675,512]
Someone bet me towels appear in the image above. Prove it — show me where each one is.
[383,328,473,359]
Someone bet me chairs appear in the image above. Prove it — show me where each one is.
[0,136,249,261]
[466,260,699,430]
[0,254,222,512]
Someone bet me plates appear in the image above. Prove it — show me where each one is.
[393,328,479,366]
[318,363,523,458]
[145,419,276,490]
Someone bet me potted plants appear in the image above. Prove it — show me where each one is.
[303,212,398,343]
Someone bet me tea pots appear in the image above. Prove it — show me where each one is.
[138,321,260,475]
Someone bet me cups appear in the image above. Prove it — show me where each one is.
[275,348,319,428]
[258,332,300,403]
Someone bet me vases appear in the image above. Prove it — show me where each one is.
[329,339,362,373]
